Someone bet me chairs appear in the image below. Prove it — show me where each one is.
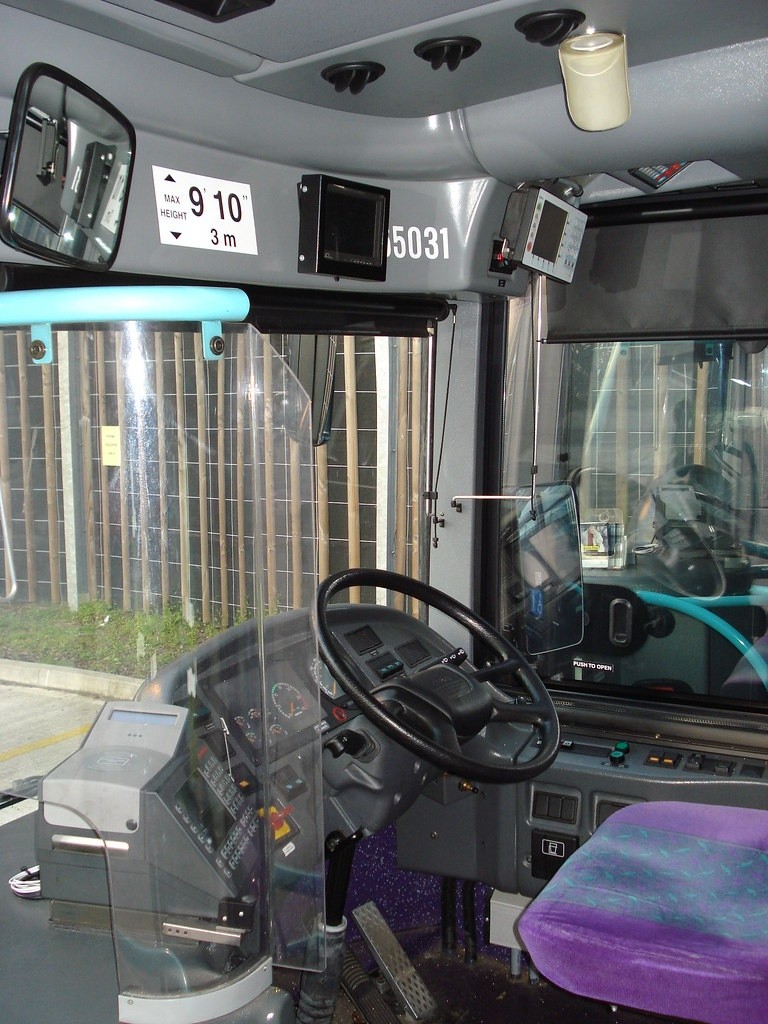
[518,800,768,1024]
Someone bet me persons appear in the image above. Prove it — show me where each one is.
[581,524,608,553]
